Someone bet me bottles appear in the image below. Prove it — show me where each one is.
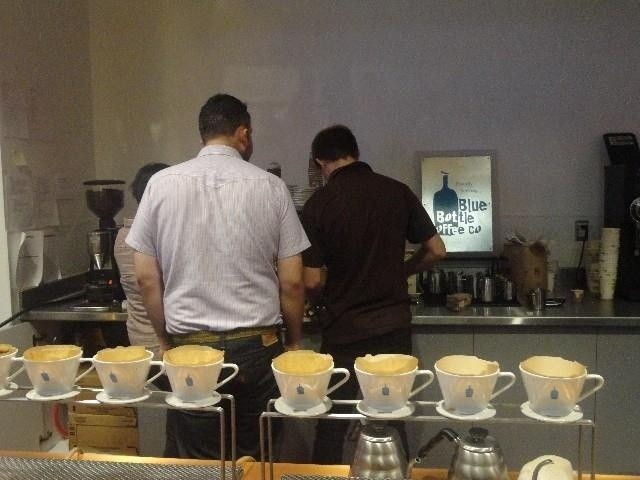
[433,172,458,235]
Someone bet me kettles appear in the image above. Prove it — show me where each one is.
[419,267,448,304]
[477,275,503,303]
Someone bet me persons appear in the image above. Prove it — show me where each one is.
[298,123,450,465]
[111,162,173,458]
[127,94,313,461]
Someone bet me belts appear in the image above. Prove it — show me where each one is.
[171,326,279,345]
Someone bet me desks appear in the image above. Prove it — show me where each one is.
[0,449,640,480]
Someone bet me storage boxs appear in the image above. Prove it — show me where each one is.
[68,372,139,455]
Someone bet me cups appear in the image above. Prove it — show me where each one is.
[23,350,96,396]
[163,354,239,401]
[0,347,25,388]
[94,351,165,398]
[528,287,545,310]
[354,361,435,412]
[308,153,327,192]
[266,163,281,181]
[518,357,605,417]
[585,226,621,301]
[435,357,517,414]
[271,359,350,410]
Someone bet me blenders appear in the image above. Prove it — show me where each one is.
[83,179,127,301]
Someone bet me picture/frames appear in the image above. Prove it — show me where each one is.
[414,149,502,260]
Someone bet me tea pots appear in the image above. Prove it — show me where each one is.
[347,424,422,480]
[420,427,553,480]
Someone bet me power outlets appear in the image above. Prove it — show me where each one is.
[575,221,589,242]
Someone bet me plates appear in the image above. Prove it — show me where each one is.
[437,399,497,421]
[0,382,19,397]
[288,185,317,213]
[27,386,83,402]
[522,400,584,424]
[273,392,333,420]
[96,390,153,405]
[356,399,417,419]
[166,391,222,410]
